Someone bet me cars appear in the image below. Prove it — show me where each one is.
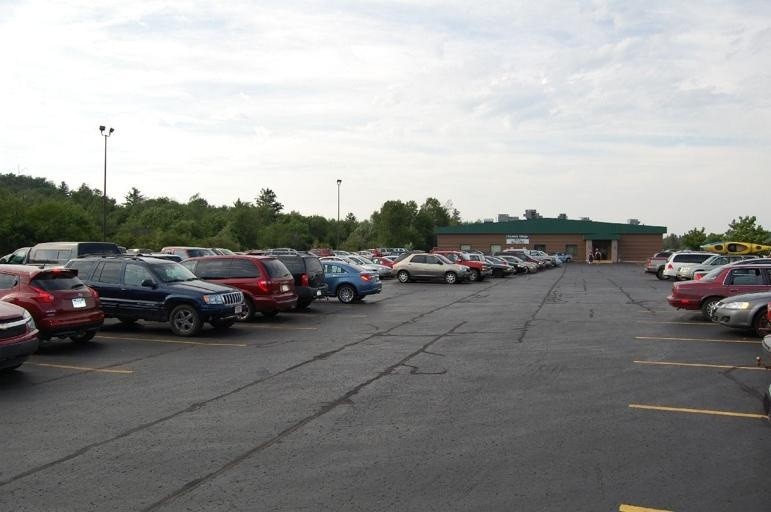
[429,248,573,282]
[644,249,771,336]
[0,262,101,343]
[117,246,426,280]
[0,246,32,264]
[390,253,472,285]
[0,297,38,370]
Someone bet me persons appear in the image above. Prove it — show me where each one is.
[587,251,595,265]
[595,248,604,263]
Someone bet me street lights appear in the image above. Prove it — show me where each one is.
[99,125,115,242]
[336,178,342,251]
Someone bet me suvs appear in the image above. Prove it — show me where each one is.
[22,241,125,263]
[66,259,245,336]
[175,254,383,321]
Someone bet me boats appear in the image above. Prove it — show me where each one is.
[699,241,771,255]
